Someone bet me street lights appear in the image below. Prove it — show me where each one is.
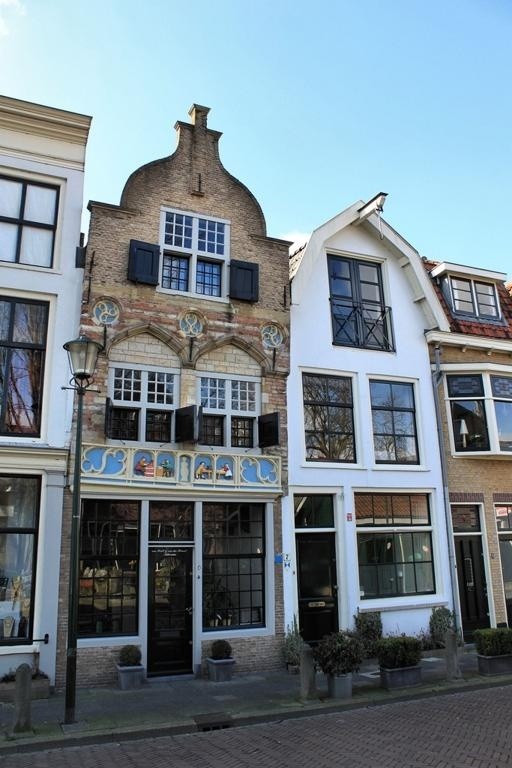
[61,332,105,726]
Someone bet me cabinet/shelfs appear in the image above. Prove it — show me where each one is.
[81,559,139,636]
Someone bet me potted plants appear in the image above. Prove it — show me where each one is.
[315,627,363,699]
[472,628,512,674]
[378,634,422,691]
[115,645,144,690]
[284,613,310,675]
[206,640,235,681]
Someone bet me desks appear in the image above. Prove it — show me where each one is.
[203,470,225,479]
